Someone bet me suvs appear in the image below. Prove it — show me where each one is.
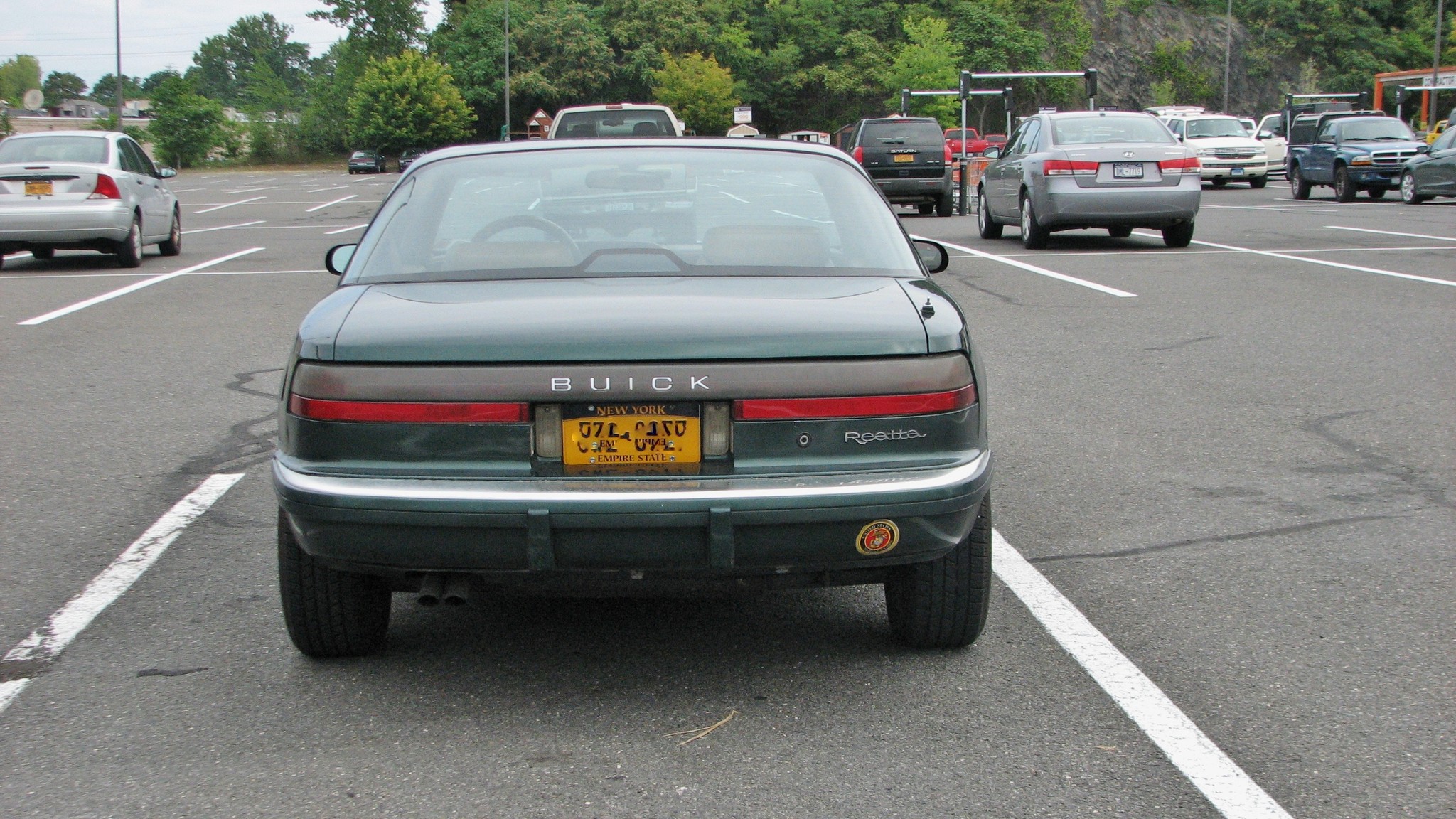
[846,116,958,217]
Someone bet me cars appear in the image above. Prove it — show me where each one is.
[398,148,429,174]
[1141,101,1456,189]
[267,136,995,660]
[1401,125,1456,205]
[976,112,1203,250]
[982,134,1008,150]
[347,150,386,174]
[0,129,183,268]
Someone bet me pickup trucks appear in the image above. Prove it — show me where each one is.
[1285,110,1428,203]
[538,101,699,247]
[943,127,989,161]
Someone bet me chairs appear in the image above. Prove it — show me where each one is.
[702,226,828,267]
[572,123,598,137]
[633,121,659,136]
[433,239,571,270]
